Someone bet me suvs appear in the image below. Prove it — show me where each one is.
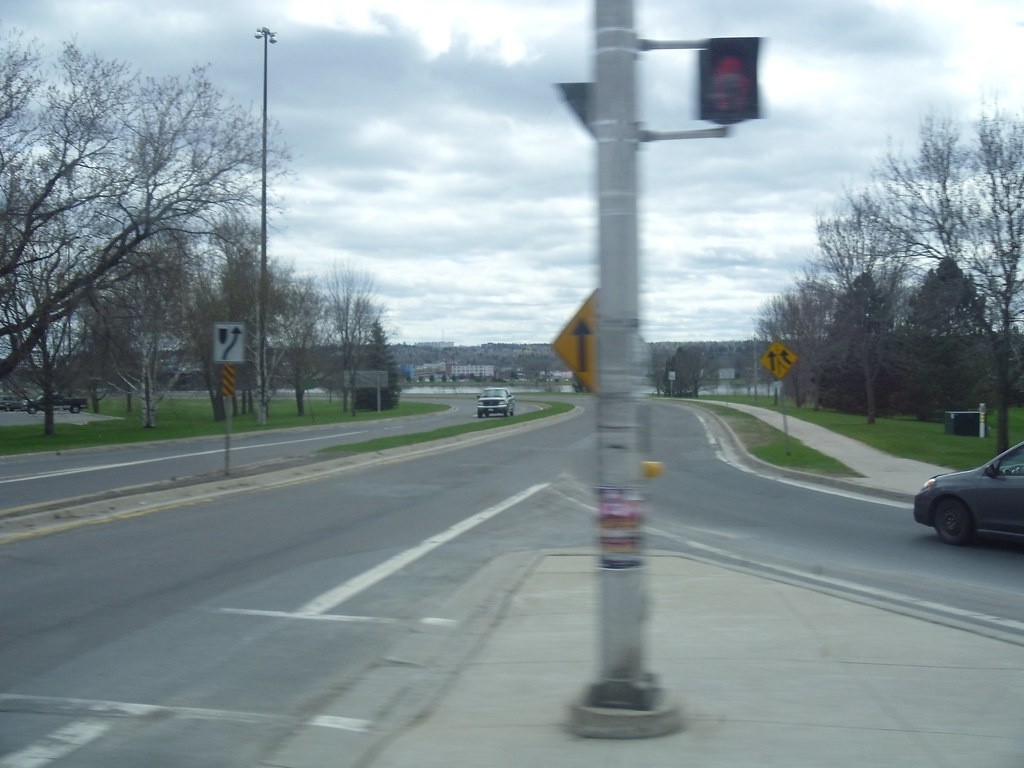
[0,395,28,412]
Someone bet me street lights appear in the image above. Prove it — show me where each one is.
[254,26,275,424]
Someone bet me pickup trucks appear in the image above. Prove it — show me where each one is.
[22,392,88,414]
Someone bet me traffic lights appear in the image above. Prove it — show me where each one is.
[697,35,760,122]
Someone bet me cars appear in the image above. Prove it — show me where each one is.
[476,388,516,419]
[914,442,1023,547]
